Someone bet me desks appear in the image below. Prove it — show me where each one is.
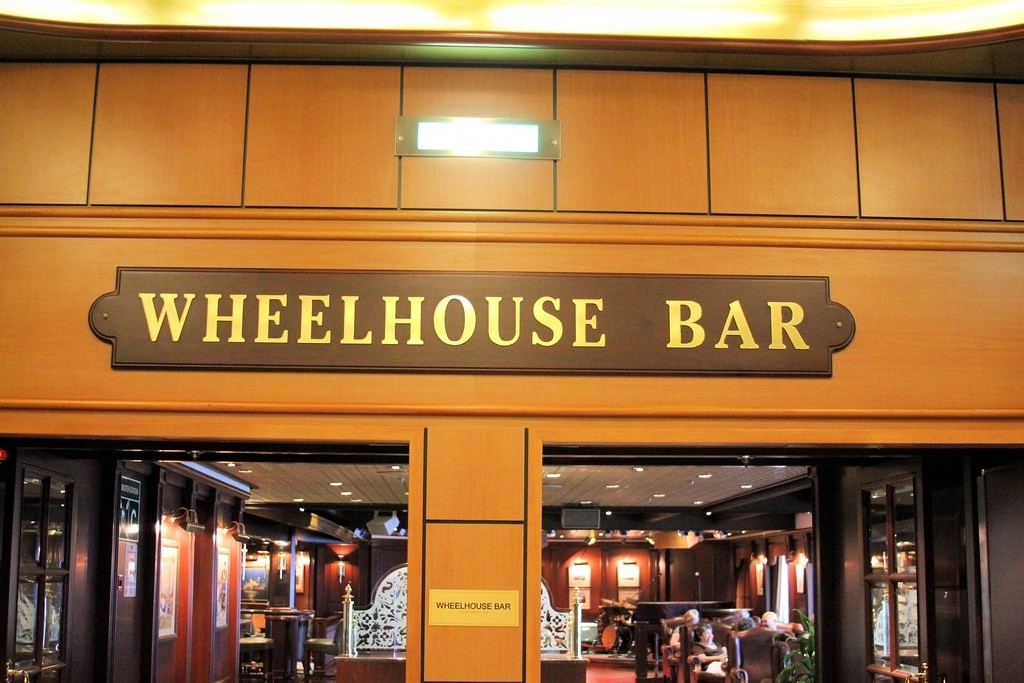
[241,608,315,682]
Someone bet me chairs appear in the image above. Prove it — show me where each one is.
[660,615,797,683]
[303,618,343,683]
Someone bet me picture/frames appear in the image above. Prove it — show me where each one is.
[241,564,269,601]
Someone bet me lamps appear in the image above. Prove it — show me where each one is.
[694,531,700,536]
[620,530,627,535]
[604,530,611,534]
[546,530,556,535]
[723,531,731,536]
[683,531,688,536]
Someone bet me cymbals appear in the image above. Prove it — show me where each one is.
[601,598,620,606]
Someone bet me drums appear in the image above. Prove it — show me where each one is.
[601,624,631,652]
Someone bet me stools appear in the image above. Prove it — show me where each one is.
[240,637,274,683]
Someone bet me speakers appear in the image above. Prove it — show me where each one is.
[367,516,400,535]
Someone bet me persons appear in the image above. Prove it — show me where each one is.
[762,611,785,634]
[737,610,756,630]
[669,609,700,650]
[691,623,728,677]
